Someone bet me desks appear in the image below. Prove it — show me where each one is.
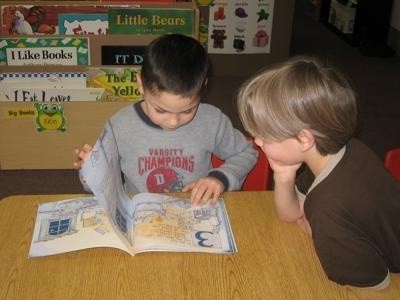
[0,190,400,300]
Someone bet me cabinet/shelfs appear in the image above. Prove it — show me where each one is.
[319,0,394,48]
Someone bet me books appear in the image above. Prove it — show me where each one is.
[26,120,238,256]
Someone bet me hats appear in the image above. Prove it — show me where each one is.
[235,8,248,18]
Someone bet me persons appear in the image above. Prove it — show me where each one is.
[72,33,260,209]
[235,54,400,290]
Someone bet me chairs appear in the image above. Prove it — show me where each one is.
[211,136,270,190]
